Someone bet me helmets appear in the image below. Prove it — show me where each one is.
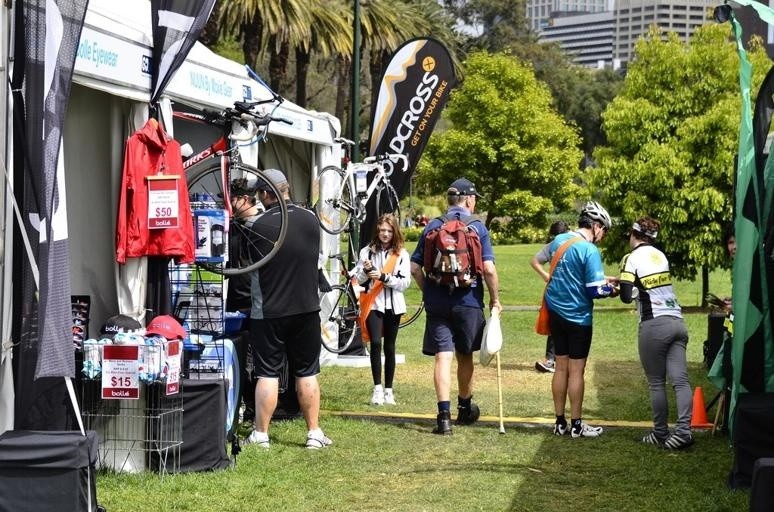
[580,199,612,228]
[145,314,189,342]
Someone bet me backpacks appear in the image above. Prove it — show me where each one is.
[422,213,482,290]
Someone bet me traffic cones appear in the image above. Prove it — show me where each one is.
[689,386,715,430]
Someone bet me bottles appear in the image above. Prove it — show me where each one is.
[208,213,226,257]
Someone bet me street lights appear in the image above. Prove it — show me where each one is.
[597,53,621,81]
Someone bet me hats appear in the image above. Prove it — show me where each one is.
[447,176,486,200]
[218,178,256,198]
[252,167,288,191]
[101,314,141,334]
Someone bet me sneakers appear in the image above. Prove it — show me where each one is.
[535,358,555,373]
[306,434,333,450]
[571,422,603,438]
[553,424,571,436]
[371,388,395,405]
[635,432,671,445]
[661,432,691,450]
[238,434,269,448]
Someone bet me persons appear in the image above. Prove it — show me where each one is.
[719,228,736,309]
[411,178,502,435]
[529,221,573,373]
[403,214,430,229]
[543,201,620,438]
[217,178,305,422]
[354,213,411,405]
[620,216,694,450]
[241,169,333,450]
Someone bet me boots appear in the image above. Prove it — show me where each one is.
[454,402,480,425]
[432,412,453,435]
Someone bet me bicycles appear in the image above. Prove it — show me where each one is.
[171,96,292,277]
[313,153,409,237]
[319,240,426,353]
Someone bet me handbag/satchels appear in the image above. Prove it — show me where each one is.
[535,296,552,336]
[480,305,503,367]
[357,292,373,341]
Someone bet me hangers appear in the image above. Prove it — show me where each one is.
[148,101,159,131]
